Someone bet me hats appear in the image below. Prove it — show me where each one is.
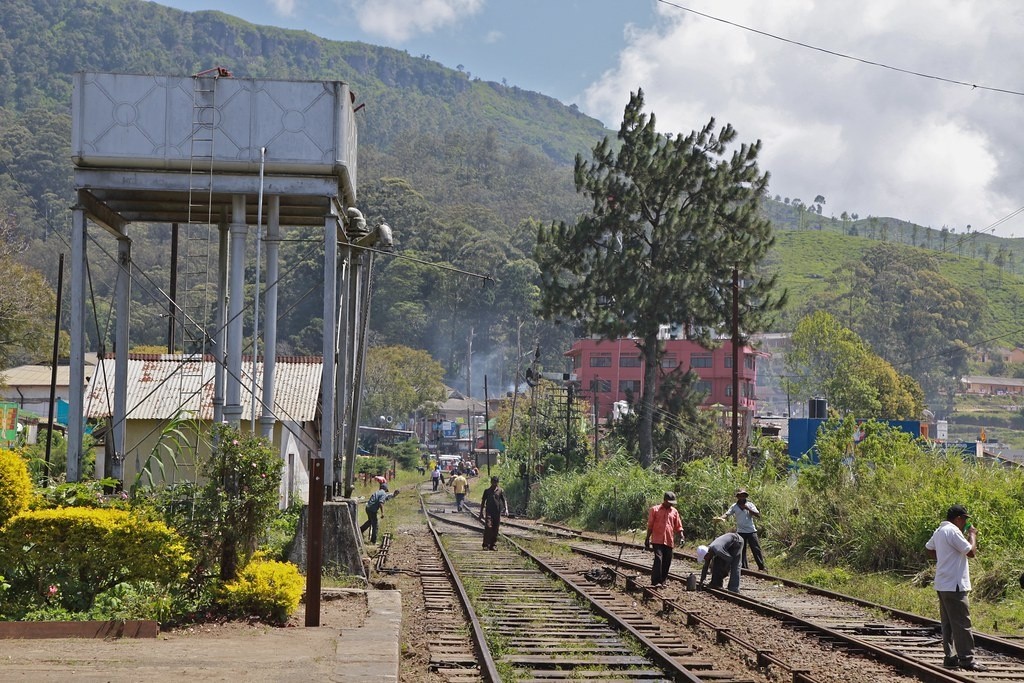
[697,545,708,561]
[664,492,677,504]
[735,488,749,496]
[948,505,973,519]
[380,484,389,492]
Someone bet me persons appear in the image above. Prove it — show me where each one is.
[453,471,468,512]
[924,506,989,672]
[386,490,399,500]
[416,466,425,476]
[446,459,478,486]
[644,492,685,590]
[478,477,509,551]
[697,533,744,593]
[372,475,389,492]
[360,484,388,545]
[430,464,445,492]
[713,488,767,570]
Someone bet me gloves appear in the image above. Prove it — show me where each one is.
[644,539,650,550]
[679,539,685,548]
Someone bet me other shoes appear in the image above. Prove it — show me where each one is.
[483,547,489,551]
[489,546,498,550]
[959,661,988,672]
[944,655,959,668]
[458,505,462,511]
